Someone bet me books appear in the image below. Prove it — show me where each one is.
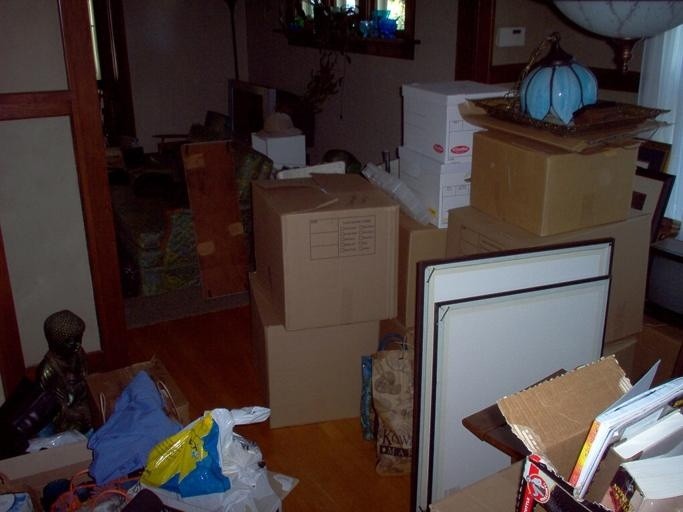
[567,358,683,512]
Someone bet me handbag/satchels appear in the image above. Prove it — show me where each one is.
[370,332,414,476]
[360,332,408,441]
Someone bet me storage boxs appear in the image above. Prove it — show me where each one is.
[396,213,447,329]
[83,353,189,427]
[250,172,400,331]
[402,79,515,164]
[247,273,381,431]
[499,353,632,512]
[470,132,639,236]
[447,205,651,343]
[398,147,473,230]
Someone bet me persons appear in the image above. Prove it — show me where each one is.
[36,309,100,430]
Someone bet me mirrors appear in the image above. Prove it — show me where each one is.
[285,0,415,60]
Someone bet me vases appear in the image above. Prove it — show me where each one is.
[369,10,396,39]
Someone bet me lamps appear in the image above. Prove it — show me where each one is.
[506,31,598,125]
[556,0,683,73]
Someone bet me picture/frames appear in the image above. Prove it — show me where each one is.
[632,167,676,242]
[426,274,612,510]
[636,136,672,172]
[410,238,616,511]
